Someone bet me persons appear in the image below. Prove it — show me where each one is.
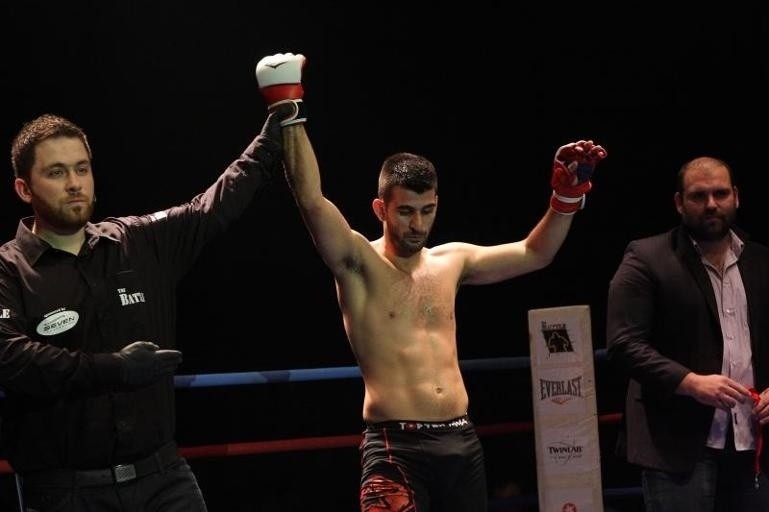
[256,49,607,510]
[608,156,767,511]
[0,104,294,512]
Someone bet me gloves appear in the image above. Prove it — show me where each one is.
[550,140,608,216]
[256,52,308,127]
[110,341,182,387]
[260,105,292,145]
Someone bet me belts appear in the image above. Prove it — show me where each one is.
[15,441,180,486]
